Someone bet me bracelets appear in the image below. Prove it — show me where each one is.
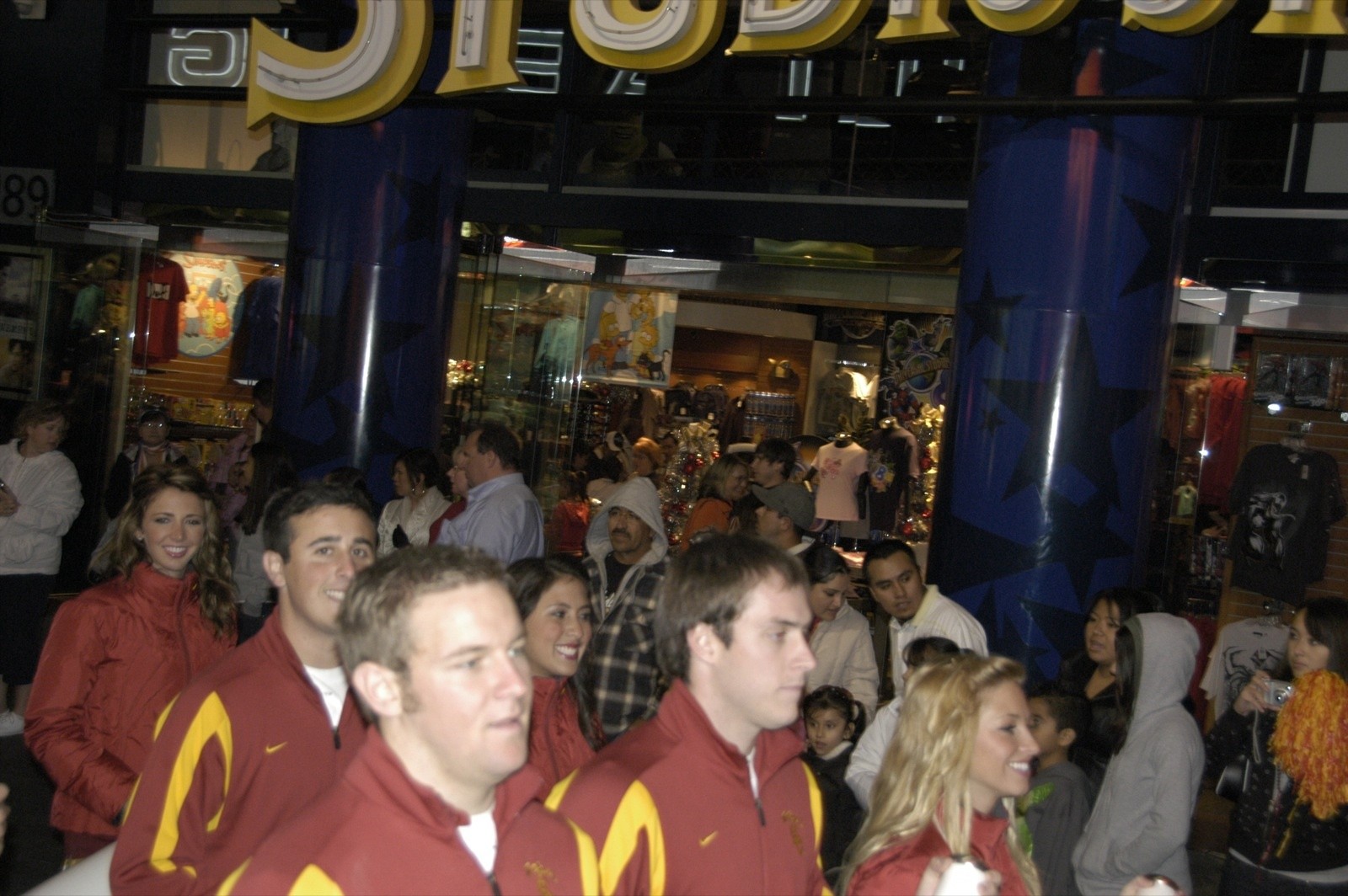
[1147,873,1179,890]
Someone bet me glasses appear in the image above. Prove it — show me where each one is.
[142,422,166,430]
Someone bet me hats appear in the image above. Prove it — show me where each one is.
[750,483,813,529]
[139,409,166,422]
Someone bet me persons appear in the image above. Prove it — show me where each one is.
[801,431,871,523]
[866,415,921,532]
[0,381,1348,896]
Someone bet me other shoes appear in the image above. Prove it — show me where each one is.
[0,710,24,736]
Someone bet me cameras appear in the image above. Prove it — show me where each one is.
[1259,679,1293,707]
